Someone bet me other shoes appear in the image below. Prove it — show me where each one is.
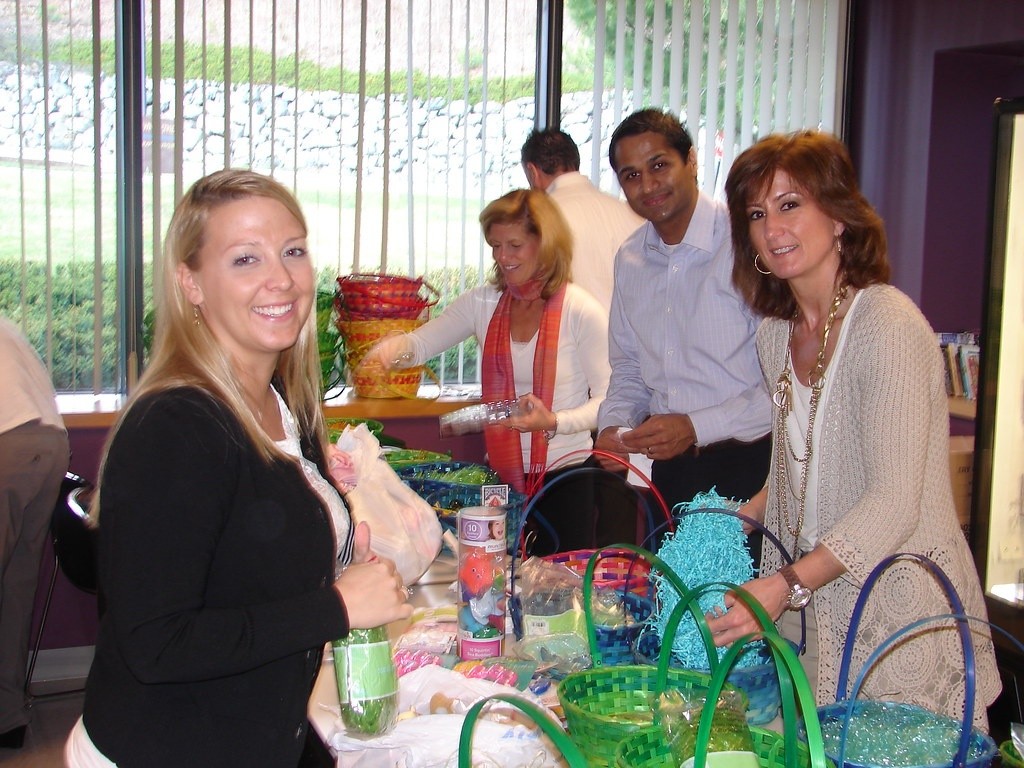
[0,722,27,747]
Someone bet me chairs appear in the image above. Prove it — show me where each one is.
[26,473,102,706]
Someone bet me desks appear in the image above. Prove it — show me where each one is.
[285,535,801,768]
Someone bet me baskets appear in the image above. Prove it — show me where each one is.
[315,269,442,402]
[324,417,1024,768]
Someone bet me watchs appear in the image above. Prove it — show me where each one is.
[777,565,812,612]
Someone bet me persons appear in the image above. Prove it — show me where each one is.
[485,521,505,543]
[57,166,416,768]
[704,129,1004,734]
[592,108,773,582]
[363,186,612,494]
[519,129,648,313]
[0,314,70,746]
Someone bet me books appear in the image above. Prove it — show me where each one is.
[934,330,980,399]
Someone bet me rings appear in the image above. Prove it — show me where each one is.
[647,447,654,454]
[402,586,414,596]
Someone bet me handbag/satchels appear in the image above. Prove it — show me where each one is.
[334,421,444,587]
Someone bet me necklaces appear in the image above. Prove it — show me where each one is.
[772,288,850,568]
